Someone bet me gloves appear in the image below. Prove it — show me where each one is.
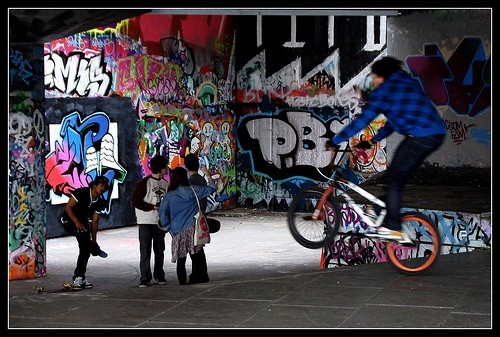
[353,141,372,149]
[325,140,335,150]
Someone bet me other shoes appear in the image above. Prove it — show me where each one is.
[364,227,403,240]
[187,277,207,284]
[179,280,187,285]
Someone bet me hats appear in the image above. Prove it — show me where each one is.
[371,57,403,80]
[89,175,110,192]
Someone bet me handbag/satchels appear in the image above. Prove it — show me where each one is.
[190,186,210,246]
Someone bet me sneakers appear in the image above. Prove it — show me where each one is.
[82,280,92,288]
[154,276,167,284]
[72,277,83,289]
[139,280,151,287]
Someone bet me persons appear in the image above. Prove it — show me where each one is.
[132,154,169,288]
[157,167,218,285]
[58,176,110,289]
[184,154,210,284]
[326,57,448,239]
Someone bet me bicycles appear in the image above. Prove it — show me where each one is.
[286,142,441,277]
